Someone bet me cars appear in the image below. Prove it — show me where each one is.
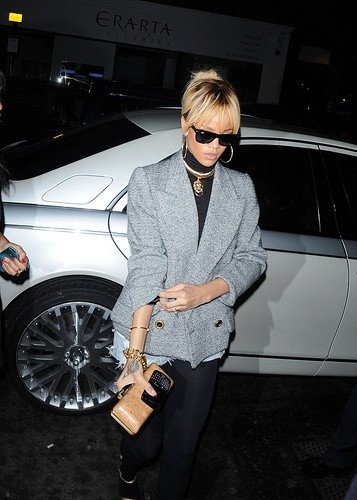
[0,105,356,416]
[0,73,356,145]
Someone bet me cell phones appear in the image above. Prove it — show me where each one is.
[0,246,20,273]
[141,370,171,410]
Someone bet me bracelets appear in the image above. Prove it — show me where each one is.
[122,326,149,372]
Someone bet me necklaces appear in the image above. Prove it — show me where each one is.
[184,160,215,196]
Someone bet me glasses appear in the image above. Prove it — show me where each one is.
[191,125,240,146]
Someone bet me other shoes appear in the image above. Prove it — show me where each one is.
[117,461,139,500]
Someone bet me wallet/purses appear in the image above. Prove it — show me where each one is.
[111,363,174,435]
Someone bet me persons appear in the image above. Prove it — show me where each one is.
[294,384,357,478]
[0,74,30,368]
[109,69,268,500]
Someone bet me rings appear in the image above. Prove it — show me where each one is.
[172,309,178,315]
[15,269,23,276]
